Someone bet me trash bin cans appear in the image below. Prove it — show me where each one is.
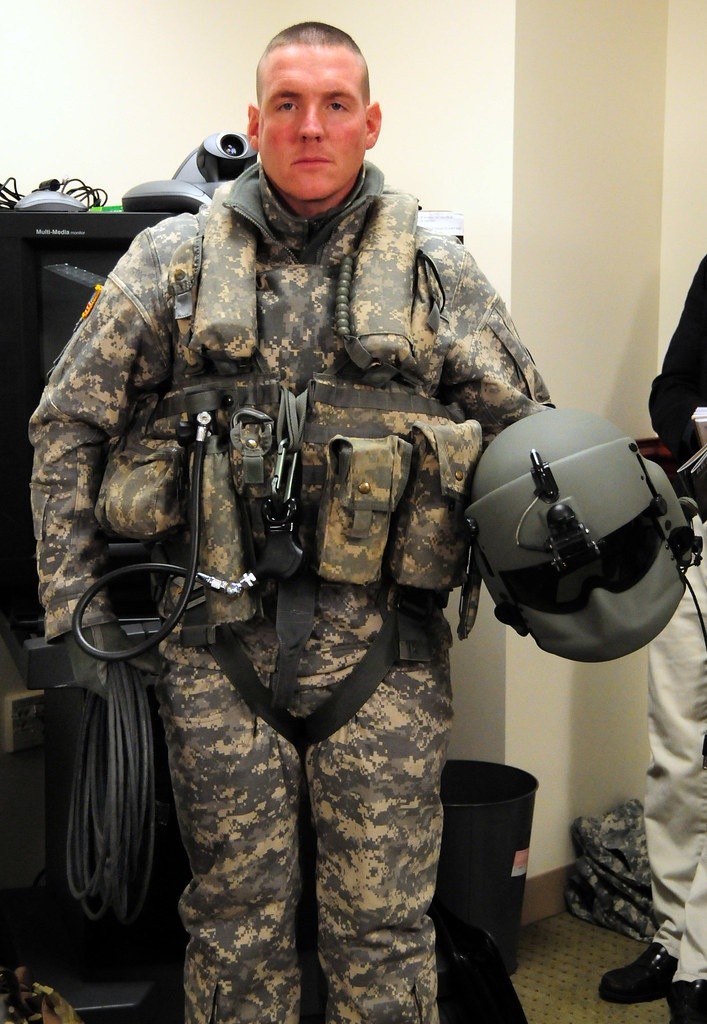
[434,758,540,976]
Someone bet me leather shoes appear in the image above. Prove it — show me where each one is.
[666,979,707,1024]
[598,943,679,1004]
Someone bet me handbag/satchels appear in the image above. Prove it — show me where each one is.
[565,798,660,943]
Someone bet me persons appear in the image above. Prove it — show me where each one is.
[599,255,707,1024]
[31,21,554,1024]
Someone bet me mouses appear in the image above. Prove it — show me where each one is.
[14,191,87,212]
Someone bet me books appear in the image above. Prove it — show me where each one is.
[676,407,707,525]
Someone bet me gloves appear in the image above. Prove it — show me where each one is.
[63,623,158,705]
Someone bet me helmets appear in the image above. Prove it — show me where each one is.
[465,408,704,662]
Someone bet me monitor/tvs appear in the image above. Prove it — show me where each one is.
[0,211,180,426]
